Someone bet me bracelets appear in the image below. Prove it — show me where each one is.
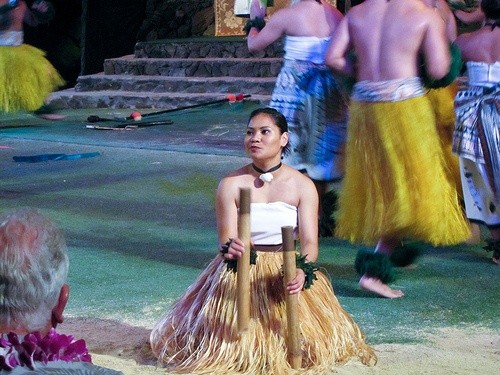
[218,237,258,274]
[280,253,321,289]
[243,15,266,35]
[417,39,463,88]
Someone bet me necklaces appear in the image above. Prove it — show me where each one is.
[484,21,500,30]
[0,327,92,370]
[250,162,282,183]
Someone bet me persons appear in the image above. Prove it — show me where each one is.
[142,106,377,375]
[247,0,500,299]
[0,0,65,120]
[0,210,93,375]
